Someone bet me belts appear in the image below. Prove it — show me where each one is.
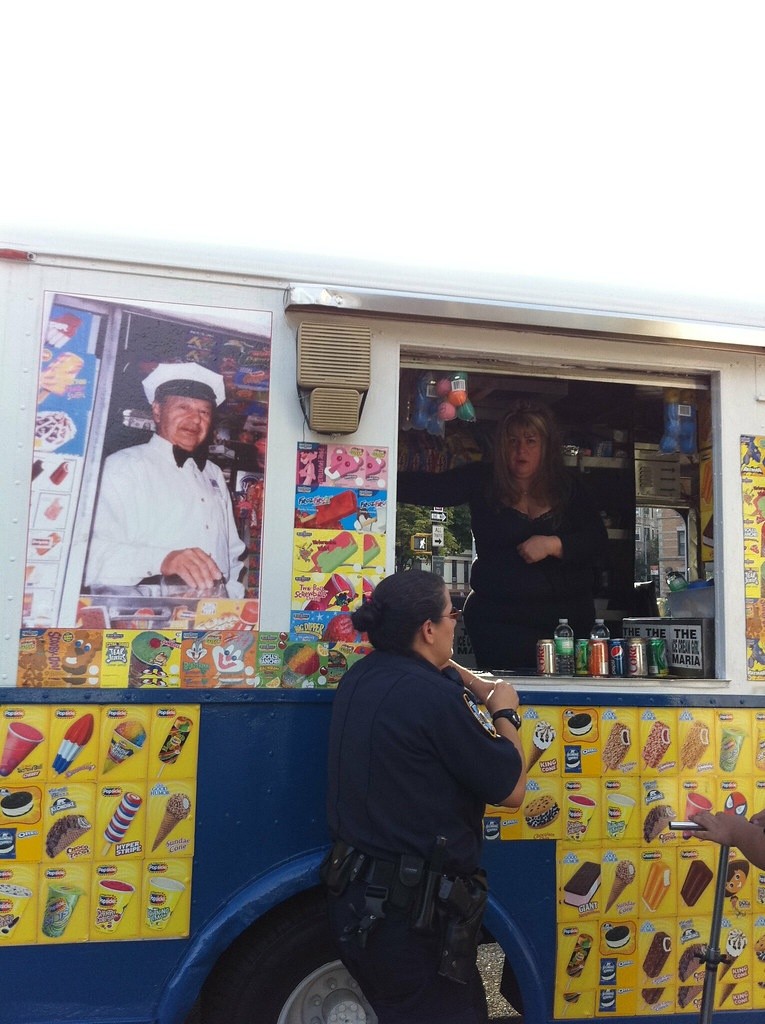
[139,573,225,587]
[355,860,393,885]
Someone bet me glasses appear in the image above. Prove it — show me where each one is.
[418,606,462,631]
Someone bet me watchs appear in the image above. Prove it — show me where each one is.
[492,708,522,730]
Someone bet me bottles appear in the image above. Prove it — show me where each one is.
[665,568,687,592]
[554,619,575,676]
[589,619,610,678]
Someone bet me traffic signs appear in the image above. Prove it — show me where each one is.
[431,512,447,522]
[432,525,445,547]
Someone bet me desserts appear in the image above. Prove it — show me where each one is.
[524,795,560,829]
[1,791,34,817]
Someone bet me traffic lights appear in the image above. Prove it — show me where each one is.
[411,535,428,553]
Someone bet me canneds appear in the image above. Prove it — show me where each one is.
[535,639,555,675]
[574,637,668,678]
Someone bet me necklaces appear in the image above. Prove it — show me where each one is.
[509,486,532,495]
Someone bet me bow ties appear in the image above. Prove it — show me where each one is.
[173,442,208,472]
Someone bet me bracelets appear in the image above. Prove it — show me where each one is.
[467,676,477,689]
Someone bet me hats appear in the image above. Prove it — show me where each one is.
[141,362,226,407]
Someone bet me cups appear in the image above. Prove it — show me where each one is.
[0,884,31,939]
[755,723,765,770]
[683,792,713,839]
[607,794,635,842]
[0,721,45,777]
[95,879,136,932]
[41,882,83,937]
[143,877,185,930]
[567,795,596,841]
[719,725,746,771]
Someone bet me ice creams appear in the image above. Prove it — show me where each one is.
[604,859,635,913]
[719,983,737,1005]
[151,793,191,852]
[34,411,77,452]
[526,721,556,774]
[719,928,748,981]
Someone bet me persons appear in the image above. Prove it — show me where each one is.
[83,361,246,598]
[316,570,527,1024]
[687,809,765,871]
[467,398,609,668]
